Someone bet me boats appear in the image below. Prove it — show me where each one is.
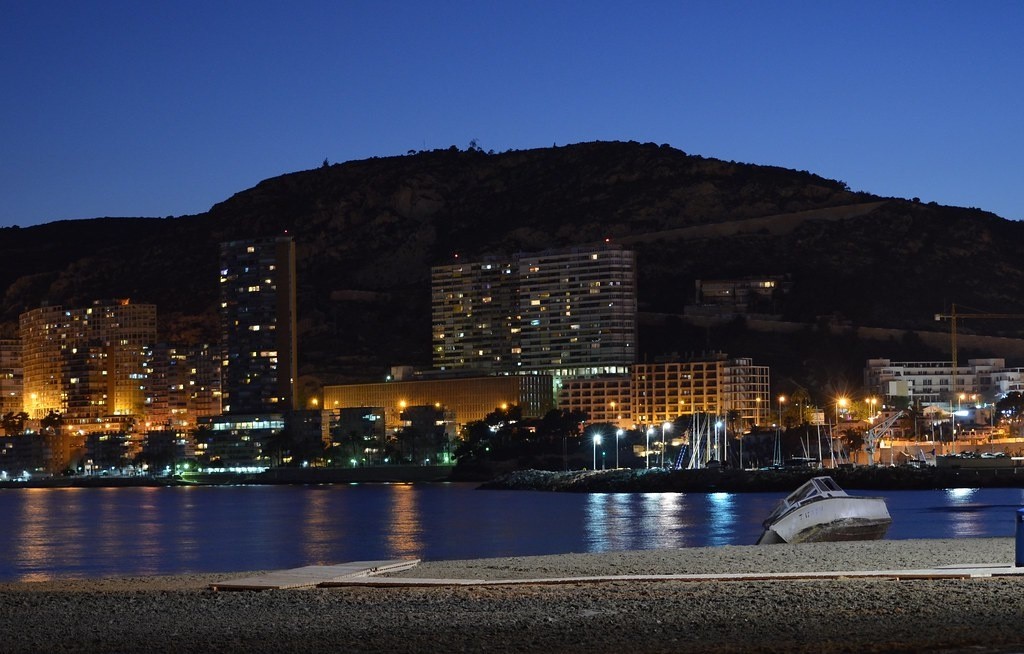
[754,475,892,545]
[181,463,283,484]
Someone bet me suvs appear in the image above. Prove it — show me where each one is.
[703,459,728,469]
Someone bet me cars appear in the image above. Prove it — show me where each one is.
[939,451,1006,459]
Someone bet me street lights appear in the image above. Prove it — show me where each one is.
[611,402,616,424]
[971,395,979,407]
[435,402,450,465]
[756,398,760,426]
[835,398,849,425]
[400,401,415,464]
[501,403,510,425]
[715,422,723,460]
[976,403,995,453]
[647,428,653,469]
[871,399,877,417]
[778,396,786,428]
[865,399,870,420]
[593,434,602,470]
[615,430,623,470]
[958,393,965,434]
[661,422,671,468]
